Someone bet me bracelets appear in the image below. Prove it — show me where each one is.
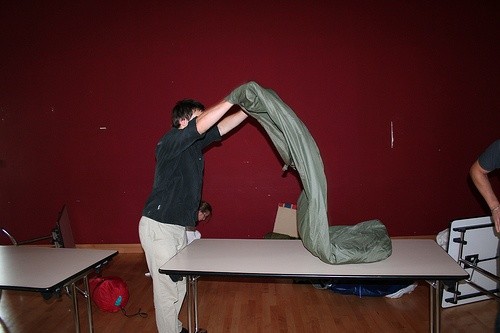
[491,206,499,212]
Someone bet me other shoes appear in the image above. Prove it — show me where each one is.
[179,328,189,333]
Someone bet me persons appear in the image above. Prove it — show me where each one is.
[185,200,212,243]
[469,141,500,331]
[139,99,250,333]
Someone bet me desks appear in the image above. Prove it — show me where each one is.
[0,204,77,248]
[440,215,500,308]
[0,245,119,333]
[158,238,471,333]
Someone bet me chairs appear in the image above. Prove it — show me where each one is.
[261,201,302,240]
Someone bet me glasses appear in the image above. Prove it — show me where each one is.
[203,211,207,219]
